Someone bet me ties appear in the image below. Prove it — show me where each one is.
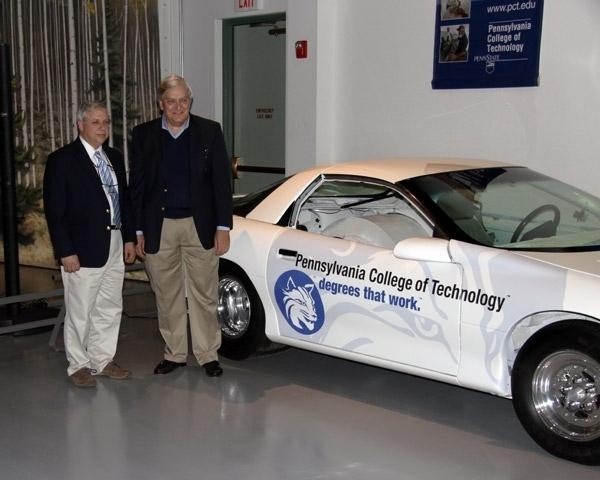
[94,151,122,229]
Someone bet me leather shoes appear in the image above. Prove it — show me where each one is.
[203,360,223,376]
[100,361,132,379]
[71,367,97,387]
[154,360,187,374]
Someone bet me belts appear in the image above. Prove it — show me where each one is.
[111,225,120,229]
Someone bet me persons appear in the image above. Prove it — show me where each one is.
[441,0,470,20]
[43,99,137,390]
[440,26,468,61]
[130,75,233,379]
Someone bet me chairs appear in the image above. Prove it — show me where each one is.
[436,191,493,246]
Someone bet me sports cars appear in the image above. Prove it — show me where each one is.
[218,155,599,468]
